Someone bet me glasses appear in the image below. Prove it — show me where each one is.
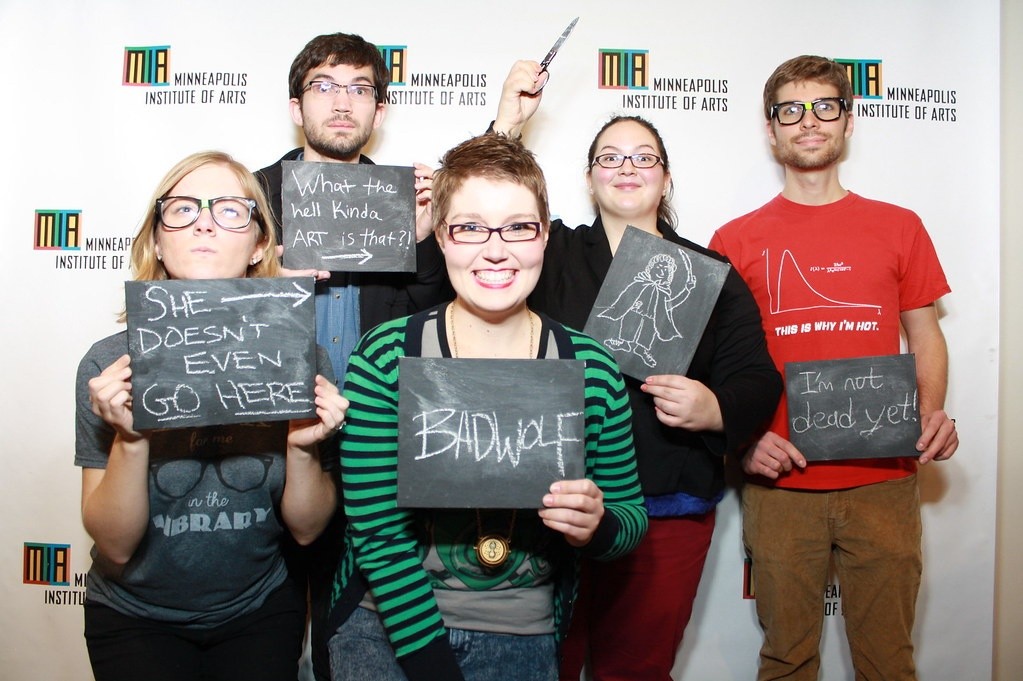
[295,80,379,103]
[440,219,545,245]
[768,97,850,126]
[153,196,266,236]
[589,152,664,169]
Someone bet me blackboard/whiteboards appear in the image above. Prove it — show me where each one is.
[581,225,732,383]
[124,276,320,432]
[780,352,924,462]
[397,354,587,510]
[277,158,418,272]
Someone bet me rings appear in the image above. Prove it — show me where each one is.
[335,420,346,431]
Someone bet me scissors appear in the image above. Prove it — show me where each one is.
[525,17,582,98]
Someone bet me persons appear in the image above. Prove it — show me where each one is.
[477,56,788,680]
[703,55,966,681]
[71,149,352,681]
[227,32,445,681]
[308,132,653,680]
